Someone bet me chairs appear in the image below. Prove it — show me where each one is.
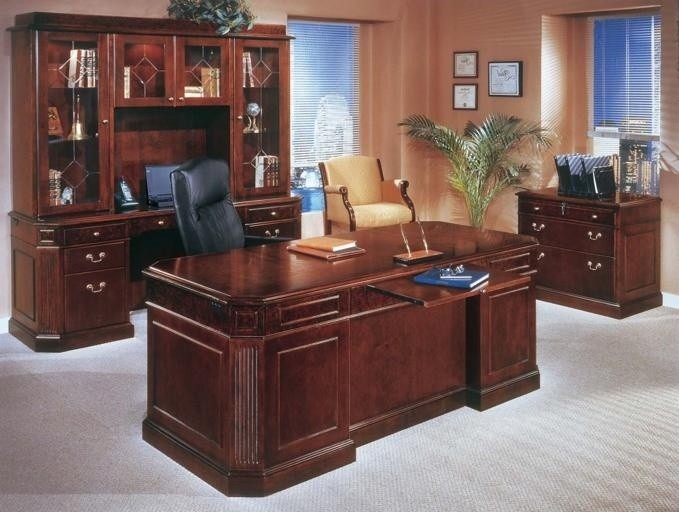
[319,156,416,236]
[171,147,298,254]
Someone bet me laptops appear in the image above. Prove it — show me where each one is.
[144,163,185,207]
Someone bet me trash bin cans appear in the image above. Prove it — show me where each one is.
[291,186,325,239]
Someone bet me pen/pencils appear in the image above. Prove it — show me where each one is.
[440,275,472,279]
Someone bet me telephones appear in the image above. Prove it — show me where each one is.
[115,176,141,208]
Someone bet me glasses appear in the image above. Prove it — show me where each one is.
[432,261,464,276]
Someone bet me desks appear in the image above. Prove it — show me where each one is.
[135,222,541,496]
[7,191,303,354]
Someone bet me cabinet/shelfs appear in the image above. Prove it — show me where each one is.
[8,219,134,352]
[518,185,662,320]
[234,195,302,244]
[11,10,295,218]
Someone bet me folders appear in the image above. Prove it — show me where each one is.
[554,154,609,195]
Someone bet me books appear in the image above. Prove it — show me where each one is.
[283,245,366,260]
[184,50,254,99]
[123,65,131,99]
[294,236,357,252]
[68,47,97,88]
[47,168,62,206]
[415,266,491,289]
[253,154,280,188]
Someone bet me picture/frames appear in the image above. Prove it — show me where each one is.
[488,62,523,97]
[453,51,479,110]
